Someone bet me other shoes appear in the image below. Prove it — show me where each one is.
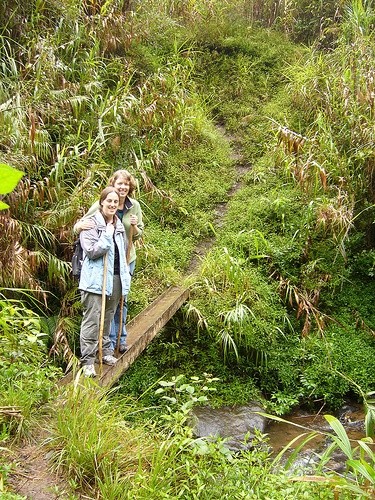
[116,345,126,353]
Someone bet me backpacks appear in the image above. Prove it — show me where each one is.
[72,239,83,275]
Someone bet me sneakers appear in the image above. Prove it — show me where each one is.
[104,354,118,365]
[83,364,96,378]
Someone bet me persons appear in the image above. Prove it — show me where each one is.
[80,187,132,378]
[73,170,144,356]
